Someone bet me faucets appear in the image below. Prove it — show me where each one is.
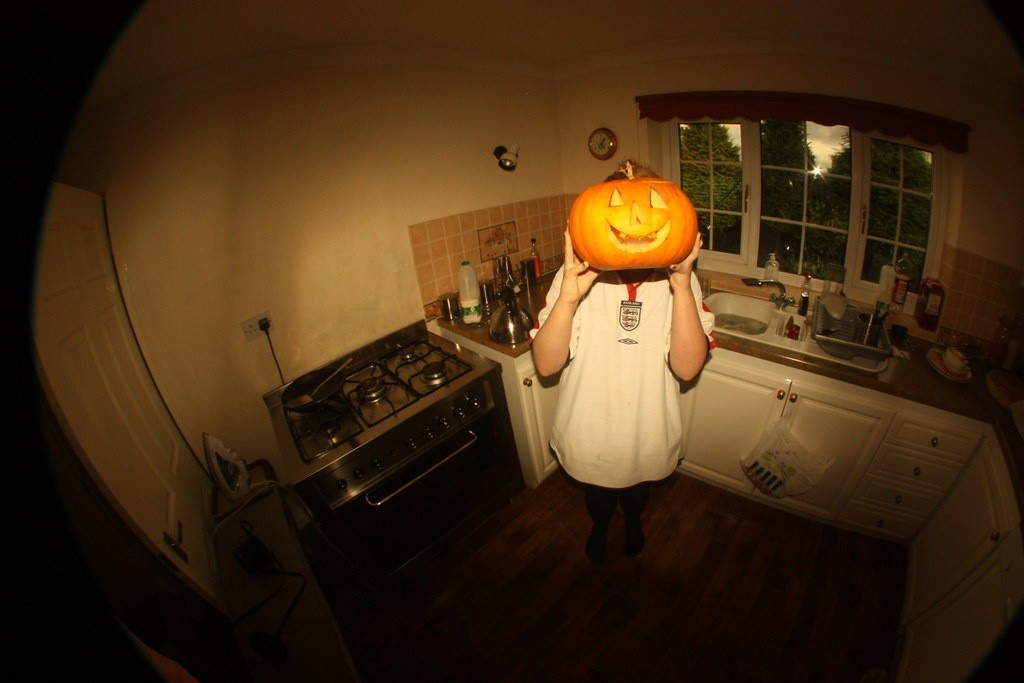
[741,278,795,312]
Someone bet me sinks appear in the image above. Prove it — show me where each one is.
[701,292,775,335]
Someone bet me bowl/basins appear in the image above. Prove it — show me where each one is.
[942,347,969,370]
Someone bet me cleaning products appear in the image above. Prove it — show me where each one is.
[764,253,779,286]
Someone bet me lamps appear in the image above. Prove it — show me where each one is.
[493,144,522,172]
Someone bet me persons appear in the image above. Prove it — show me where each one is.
[528,157,716,563]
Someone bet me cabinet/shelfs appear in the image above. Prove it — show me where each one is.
[904,437,1021,683]
[440,326,559,490]
[682,348,895,523]
[835,410,984,543]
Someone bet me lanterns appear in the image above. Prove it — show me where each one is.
[567,160,698,271]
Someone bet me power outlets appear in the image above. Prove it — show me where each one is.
[239,310,275,343]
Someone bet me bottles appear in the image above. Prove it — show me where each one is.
[438,293,459,319]
[798,272,812,316]
[876,252,913,318]
[530,238,541,287]
[459,262,483,324]
[988,315,1024,372]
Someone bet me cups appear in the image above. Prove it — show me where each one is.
[698,271,711,299]
[519,258,537,287]
[856,311,882,347]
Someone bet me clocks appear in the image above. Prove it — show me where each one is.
[588,127,617,160]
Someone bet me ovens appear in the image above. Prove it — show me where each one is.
[315,389,523,576]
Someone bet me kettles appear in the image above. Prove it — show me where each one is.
[490,286,533,345]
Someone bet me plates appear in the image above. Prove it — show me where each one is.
[926,347,972,382]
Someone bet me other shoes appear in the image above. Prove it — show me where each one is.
[624,513,644,557]
[586,521,607,564]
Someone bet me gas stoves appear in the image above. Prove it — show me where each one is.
[265,339,502,515]
